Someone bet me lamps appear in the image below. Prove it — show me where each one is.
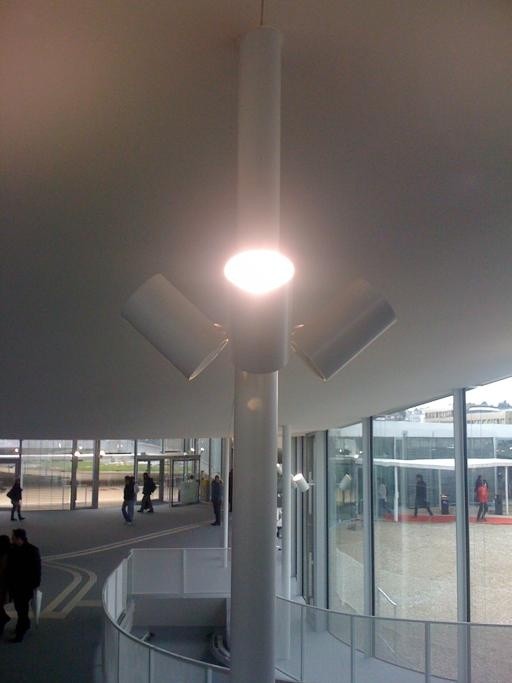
[280,275,399,382]
[117,273,230,379]
[276,463,282,475]
[338,472,352,492]
[224,245,295,375]
[293,473,310,492]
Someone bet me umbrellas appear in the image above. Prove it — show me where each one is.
[31,588,43,630]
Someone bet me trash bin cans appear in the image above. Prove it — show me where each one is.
[495,495,502,515]
[441,496,449,514]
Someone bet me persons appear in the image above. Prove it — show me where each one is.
[121,468,232,525]
[376,473,502,521]
[7,478,25,521]
[1,534,14,626]
[9,528,42,643]
